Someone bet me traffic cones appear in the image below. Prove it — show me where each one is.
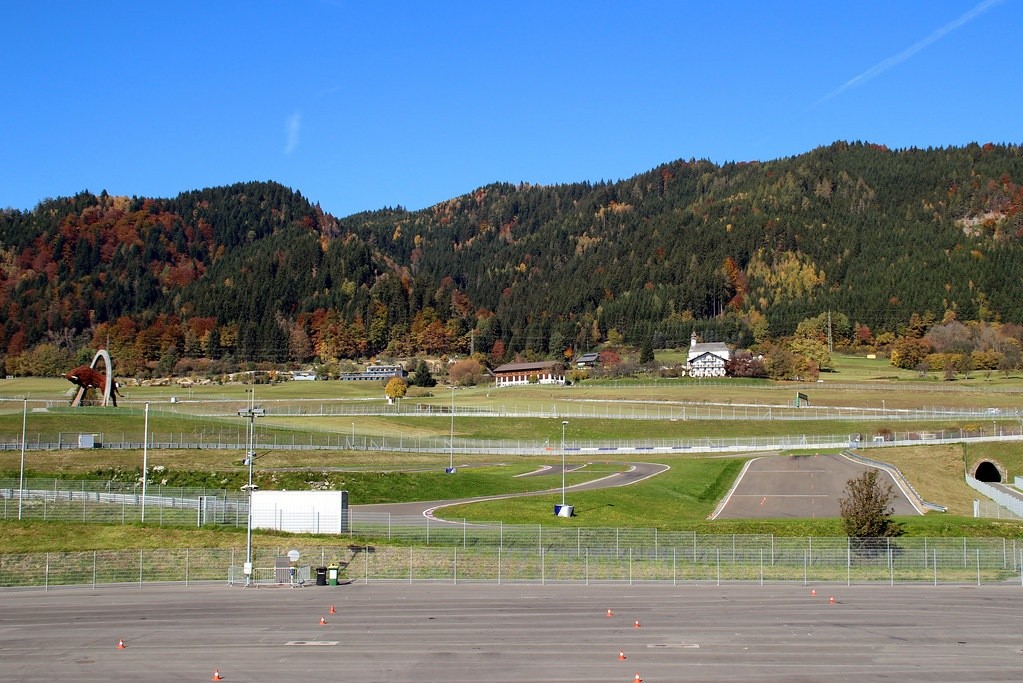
[211,668,222,681]
[606,608,614,617]
[318,616,327,625]
[330,605,335,614]
[117,638,125,649]
[828,595,835,603]
[619,649,626,660]
[634,620,641,629]
[811,589,817,596]
[633,671,642,683]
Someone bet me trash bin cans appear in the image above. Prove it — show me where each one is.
[327,566,339,586]
[316,567,327,586]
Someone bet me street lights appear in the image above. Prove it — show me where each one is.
[450,385,459,469]
[141,401,149,523]
[561,421,569,506]
[17,397,28,520]
